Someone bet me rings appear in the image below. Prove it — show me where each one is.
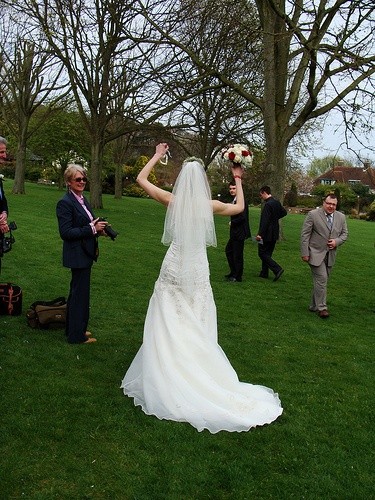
[4,217,7,220]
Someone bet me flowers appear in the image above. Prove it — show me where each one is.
[221,143,254,169]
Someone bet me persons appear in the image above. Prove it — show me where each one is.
[0,135,10,257]
[256,185,287,281]
[56,164,112,345]
[223,181,251,283]
[300,194,349,318]
[119,143,284,435]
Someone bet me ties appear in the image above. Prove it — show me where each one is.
[81,200,93,222]
[326,215,332,229]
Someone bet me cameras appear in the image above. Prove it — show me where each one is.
[7,222,17,230]
[93,216,119,241]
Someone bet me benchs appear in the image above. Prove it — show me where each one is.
[37,179,52,186]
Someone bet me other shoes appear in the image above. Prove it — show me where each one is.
[259,271,269,278]
[225,274,242,282]
[83,338,96,344]
[320,310,329,318]
[86,331,91,336]
[273,269,285,281]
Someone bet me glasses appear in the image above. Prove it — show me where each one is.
[71,177,87,182]
[324,200,336,206]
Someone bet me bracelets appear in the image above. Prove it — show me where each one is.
[233,175,241,179]
